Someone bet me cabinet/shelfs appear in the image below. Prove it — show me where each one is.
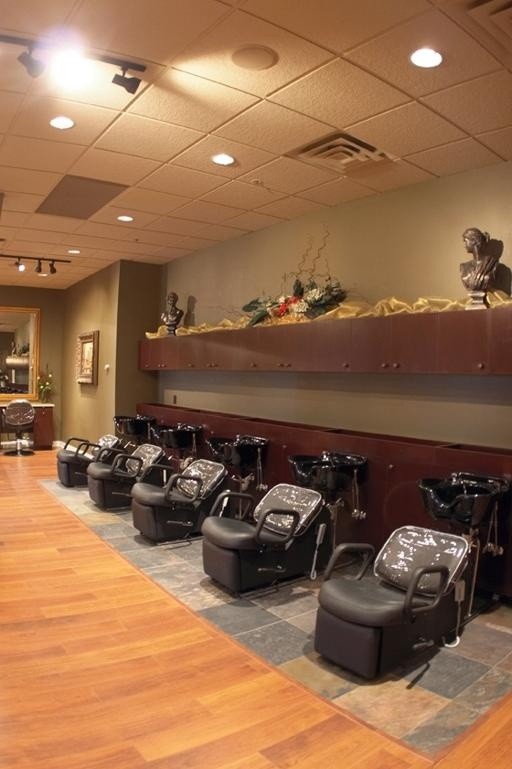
[0,403,55,447]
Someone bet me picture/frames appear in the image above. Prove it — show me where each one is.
[76,330,99,385]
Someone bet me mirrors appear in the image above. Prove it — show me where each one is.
[0,306,41,401]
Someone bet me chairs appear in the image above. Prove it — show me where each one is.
[0,400,37,455]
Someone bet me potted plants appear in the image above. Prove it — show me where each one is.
[36,363,55,404]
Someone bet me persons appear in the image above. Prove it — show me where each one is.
[160,291,183,336]
[460,227,499,289]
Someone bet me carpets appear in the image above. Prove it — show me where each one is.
[39,478,512,765]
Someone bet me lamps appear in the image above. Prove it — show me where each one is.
[0,35,147,95]
[0,255,71,274]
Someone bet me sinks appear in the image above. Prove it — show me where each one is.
[416,475,508,528]
[205,434,270,465]
[150,424,200,449]
[288,452,367,493]
[113,414,156,435]
